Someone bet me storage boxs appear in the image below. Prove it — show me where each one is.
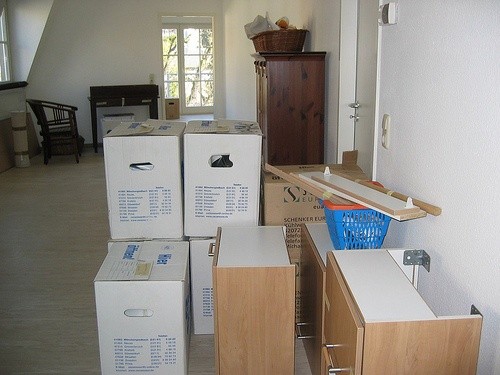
[262,166,373,325]
[166,99,180,121]
[94,120,267,374]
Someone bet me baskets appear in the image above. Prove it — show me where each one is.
[249,30,309,53]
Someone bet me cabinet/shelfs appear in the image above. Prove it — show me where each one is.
[295,223,386,375]
[252,50,327,165]
[208,224,298,375]
[324,248,482,375]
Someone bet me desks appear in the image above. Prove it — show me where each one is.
[86,82,161,152]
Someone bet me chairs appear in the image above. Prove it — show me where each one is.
[24,98,84,162]
